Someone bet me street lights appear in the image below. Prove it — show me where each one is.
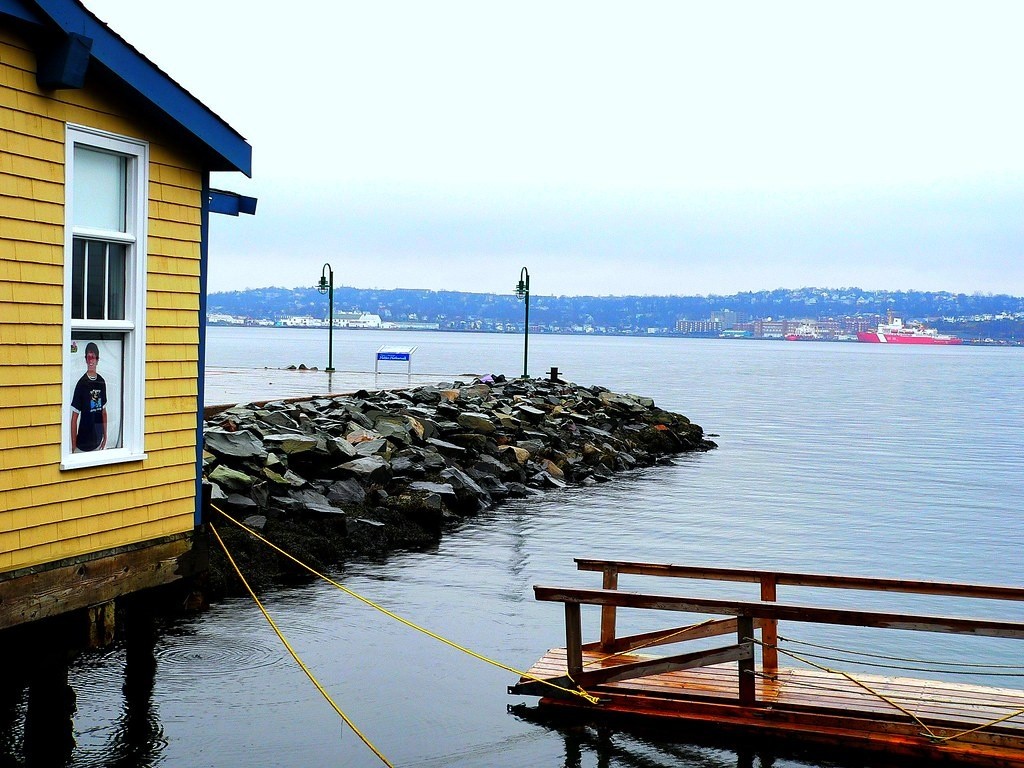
[317,264,337,374]
[514,267,532,379]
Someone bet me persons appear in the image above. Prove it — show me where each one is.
[71,342,107,453]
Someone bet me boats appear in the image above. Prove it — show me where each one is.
[718,329,750,339]
[857,317,964,347]
